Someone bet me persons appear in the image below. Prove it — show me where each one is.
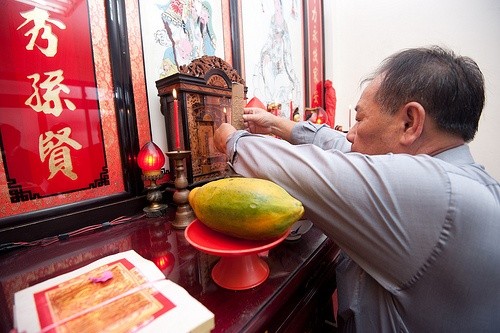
[214,46,500,333]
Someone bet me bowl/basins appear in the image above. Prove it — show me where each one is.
[285,219,313,240]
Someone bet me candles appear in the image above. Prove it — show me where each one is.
[172,89,180,149]
[290,100,293,120]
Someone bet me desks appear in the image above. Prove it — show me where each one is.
[0,205,349,333]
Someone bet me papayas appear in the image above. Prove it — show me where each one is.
[188,177,305,241]
